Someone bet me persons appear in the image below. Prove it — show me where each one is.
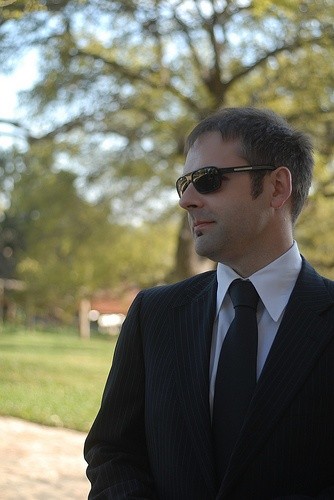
[83,105,334,500]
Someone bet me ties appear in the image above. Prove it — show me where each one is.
[210,279,260,485]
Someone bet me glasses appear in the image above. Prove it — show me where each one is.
[176,165,278,198]
